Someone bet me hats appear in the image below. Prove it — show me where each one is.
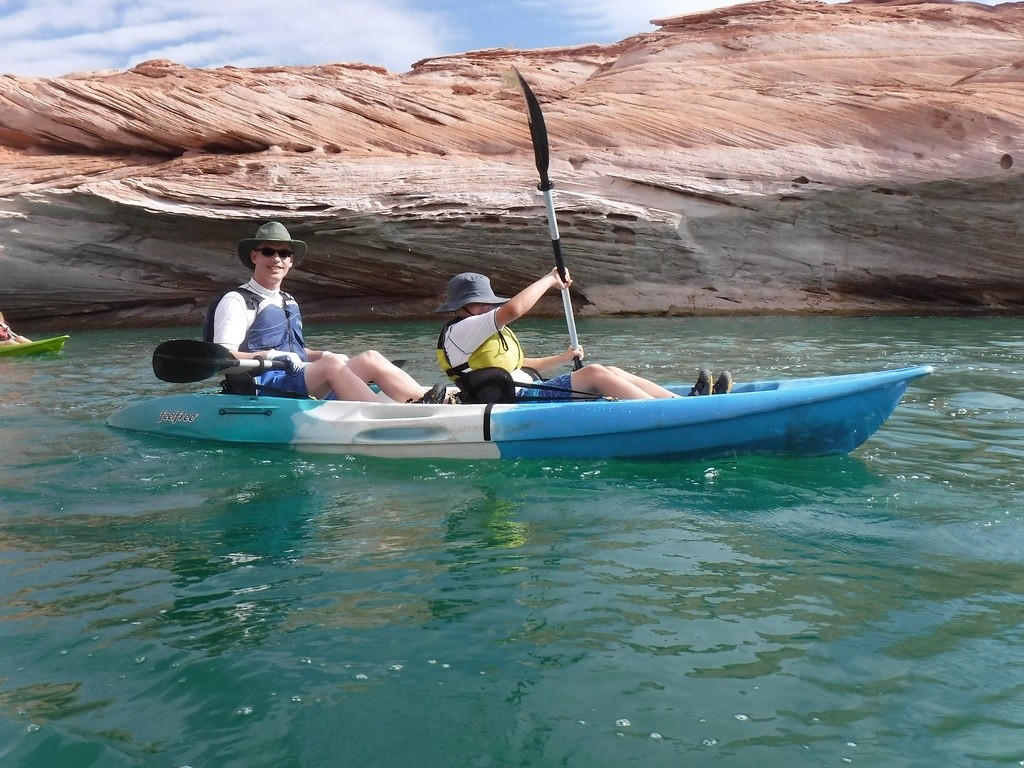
[433,273,511,313]
[238,222,307,271]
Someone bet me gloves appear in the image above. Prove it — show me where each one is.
[266,348,304,376]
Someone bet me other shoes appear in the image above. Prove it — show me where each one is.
[687,369,713,396]
[713,371,733,394]
[412,382,447,404]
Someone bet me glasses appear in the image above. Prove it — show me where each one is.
[253,248,294,258]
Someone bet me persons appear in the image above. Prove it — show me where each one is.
[436,266,733,401]
[0,313,32,345]
[214,221,450,403]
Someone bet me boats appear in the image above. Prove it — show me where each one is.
[101,359,936,462]
[0,335,70,359]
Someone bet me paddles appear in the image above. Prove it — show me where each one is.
[152,339,408,384]
[509,62,584,372]
